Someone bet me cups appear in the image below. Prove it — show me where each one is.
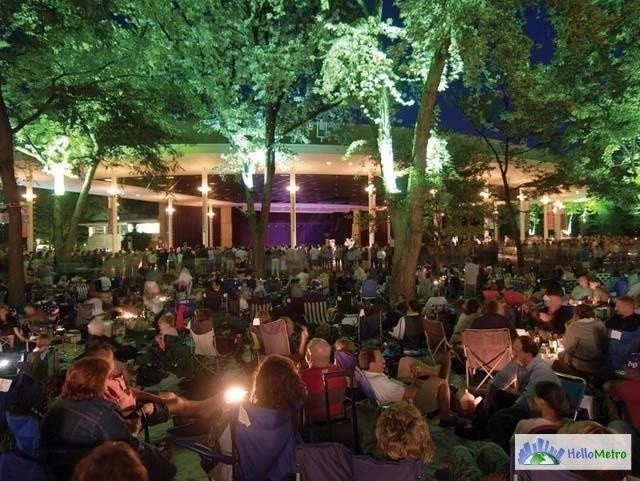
[532,325,565,354]
[409,361,420,381]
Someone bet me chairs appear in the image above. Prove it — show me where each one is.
[0,230,640,481]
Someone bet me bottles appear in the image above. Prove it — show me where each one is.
[459,388,475,419]
[55,327,66,341]
[37,331,50,347]
